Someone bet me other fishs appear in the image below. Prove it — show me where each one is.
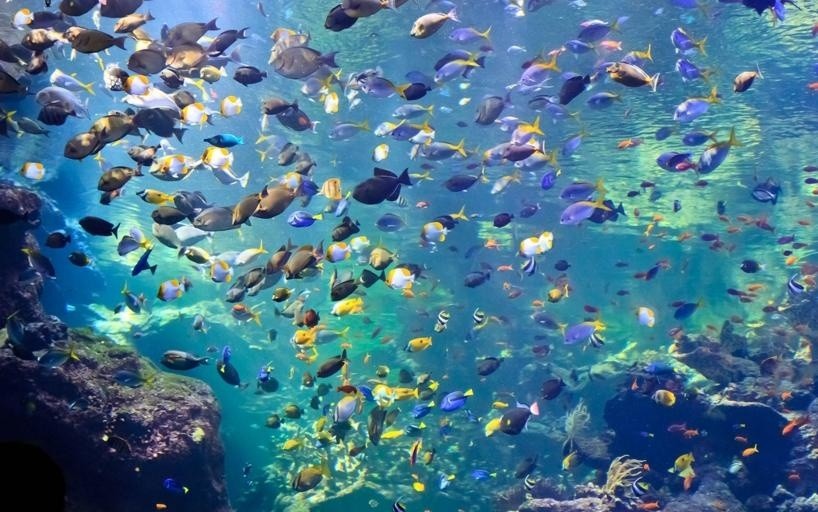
[1,0,817,512]
